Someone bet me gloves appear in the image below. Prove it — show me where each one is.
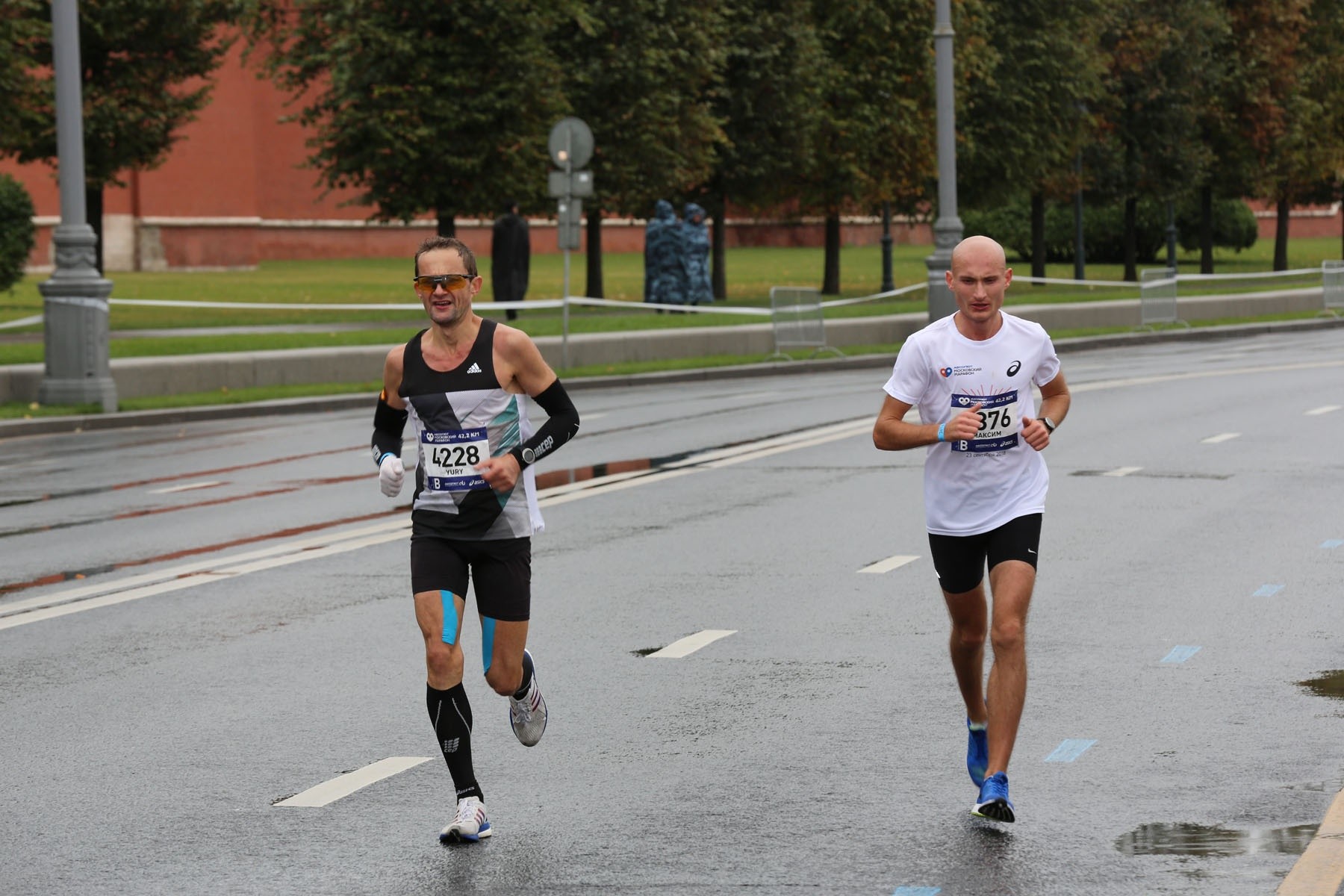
[380,456,405,498]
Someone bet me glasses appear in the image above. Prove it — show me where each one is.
[413,274,474,292]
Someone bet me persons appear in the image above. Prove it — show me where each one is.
[644,202,713,313]
[490,197,532,322]
[874,235,1071,822]
[370,237,580,843]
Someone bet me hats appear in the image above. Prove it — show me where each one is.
[685,204,705,219]
[655,200,673,218]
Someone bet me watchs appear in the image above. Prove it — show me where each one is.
[1036,416,1055,435]
[514,445,535,465]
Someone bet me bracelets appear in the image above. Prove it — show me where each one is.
[378,452,396,469]
[938,423,946,443]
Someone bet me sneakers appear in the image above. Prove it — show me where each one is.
[509,649,548,747]
[966,698,988,788]
[441,796,492,842]
[973,770,1016,823]
